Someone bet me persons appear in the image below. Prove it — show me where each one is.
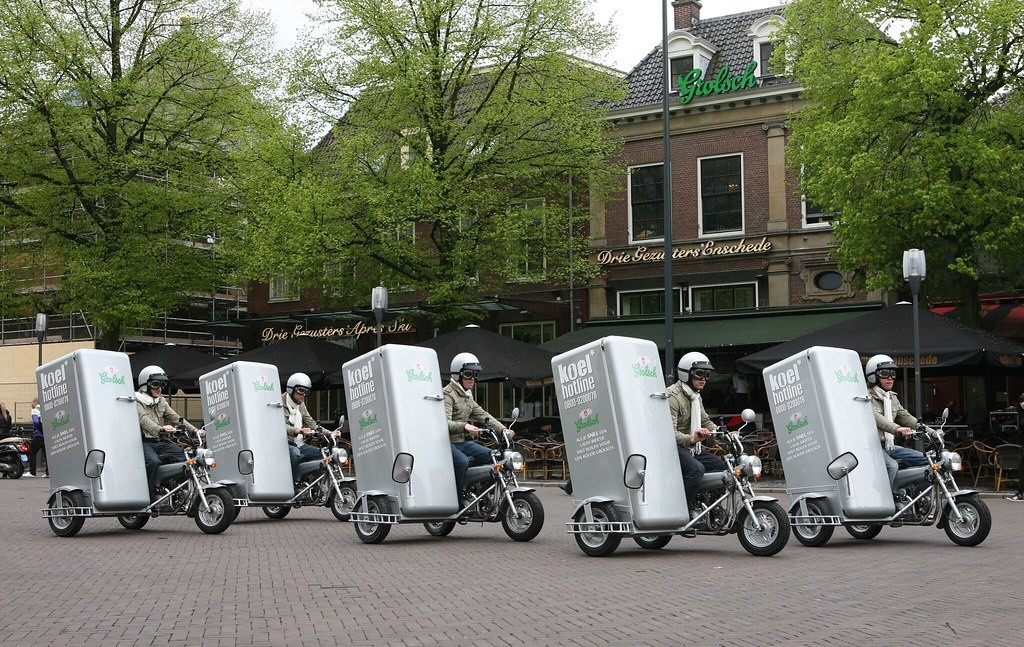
[666,351,738,524]
[1006,392,1024,502]
[865,355,943,499]
[282,373,341,485]
[718,384,757,431]
[558,479,573,496]
[22,400,49,478]
[0,402,12,478]
[443,352,514,515]
[133,365,206,491]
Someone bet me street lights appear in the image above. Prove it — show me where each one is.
[902,247,925,456]
[372,286,388,348]
[34,313,47,367]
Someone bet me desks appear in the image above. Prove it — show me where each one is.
[926,425,968,431]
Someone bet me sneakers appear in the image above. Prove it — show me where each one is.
[1006,493,1024,502]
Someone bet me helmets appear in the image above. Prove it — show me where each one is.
[865,354,897,383]
[139,365,169,392]
[451,352,482,381]
[677,352,714,382]
[286,372,311,395]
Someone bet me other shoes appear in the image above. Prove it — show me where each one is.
[22,472,35,477]
[151,491,162,510]
[42,474,49,478]
[558,485,570,495]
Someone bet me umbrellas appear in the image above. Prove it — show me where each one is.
[130,345,225,407]
[167,335,366,395]
[738,295,1024,408]
[415,323,559,409]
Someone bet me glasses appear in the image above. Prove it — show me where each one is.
[295,387,310,396]
[461,370,480,380]
[691,369,710,381]
[150,380,167,390]
[876,369,896,379]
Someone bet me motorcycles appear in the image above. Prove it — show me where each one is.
[767,343,993,548]
[0,437,34,479]
[550,335,792,559]
[199,361,358,522]
[34,348,235,537]
[340,344,544,545]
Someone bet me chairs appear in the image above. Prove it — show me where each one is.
[700,430,782,483]
[483,439,568,481]
[902,433,1024,493]
[303,432,353,477]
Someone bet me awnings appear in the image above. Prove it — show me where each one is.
[537,307,881,352]
